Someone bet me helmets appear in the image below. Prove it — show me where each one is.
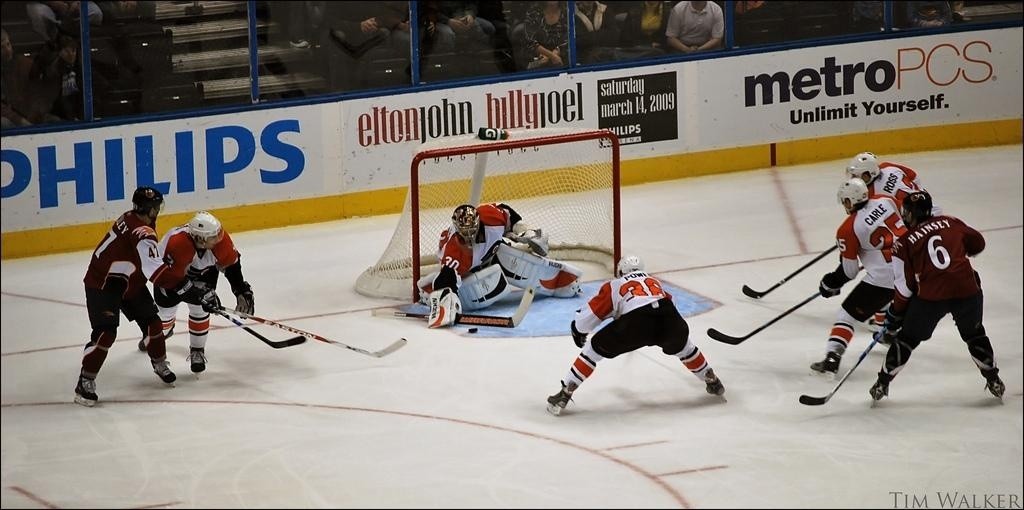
[189,211,224,251]
[845,151,882,187]
[451,204,480,248]
[617,255,644,278]
[902,192,932,229]
[131,187,163,216]
[836,178,869,215]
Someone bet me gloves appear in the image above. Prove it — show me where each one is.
[570,320,587,348]
[199,285,221,314]
[819,272,842,298]
[173,278,203,305]
[883,306,901,328]
[231,281,254,319]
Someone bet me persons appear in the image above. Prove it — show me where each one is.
[870,192,1006,405]
[415,202,582,329]
[790,0,965,43]
[808,176,907,377]
[76,186,178,402]
[578,1,789,61]
[2,2,205,126]
[150,211,254,381]
[290,1,569,79]
[546,257,725,416]
[846,150,944,227]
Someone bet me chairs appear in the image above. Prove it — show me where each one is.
[1,0,203,129]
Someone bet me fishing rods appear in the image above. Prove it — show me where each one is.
[199,298,306,348]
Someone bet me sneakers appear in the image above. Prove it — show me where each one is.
[547,384,574,411]
[139,326,172,350]
[703,377,724,396]
[870,383,887,401]
[873,332,895,344]
[288,39,307,48]
[987,377,1005,396]
[185,347,207,372]
[810,352,841,375]
[151,361,175,383]
[74,377,97,401]
